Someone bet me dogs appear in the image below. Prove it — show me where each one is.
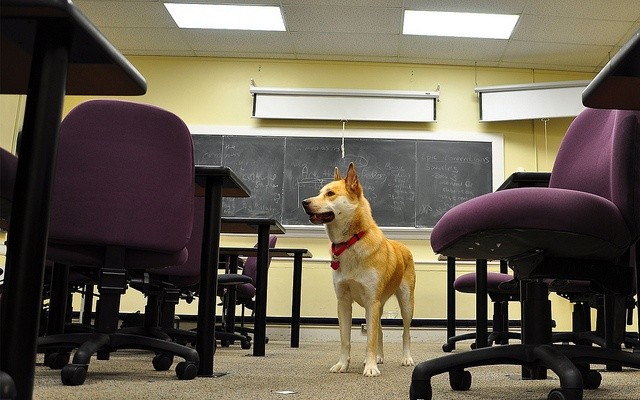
[302,162,416,377]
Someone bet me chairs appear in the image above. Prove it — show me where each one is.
[411,108,640,399]
[443,273,520,352]
[36,100,200,386]
[0,146,20,233]
[217,273,252,349]
[114,187,205,346]
[223,236,277,344]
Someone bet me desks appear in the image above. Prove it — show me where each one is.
[0,163,252,377]
[217,249,313,348]
[499,254,509,345]
[218,217,286,357]
[1,0,147,400]
[494,172,640,379]
